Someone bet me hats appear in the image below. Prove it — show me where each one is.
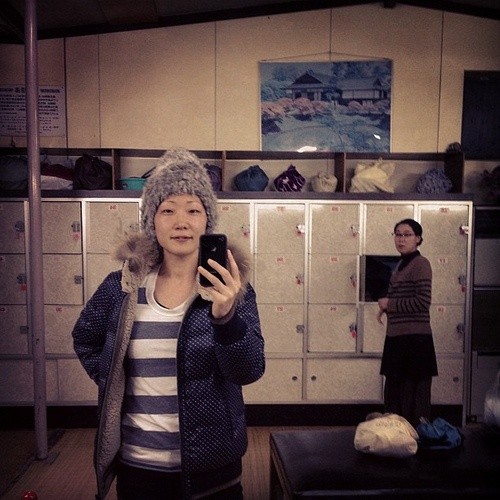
[138,145,219,240]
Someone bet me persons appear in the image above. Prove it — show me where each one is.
[376,218,439,425]
[70,142,266,500]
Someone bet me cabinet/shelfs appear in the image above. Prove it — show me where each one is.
[465,160,500,423]
[1,199,474,428]
[1,142,465,200]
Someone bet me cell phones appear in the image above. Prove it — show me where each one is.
[200,233,228,286]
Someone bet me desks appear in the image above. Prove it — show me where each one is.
[269,428,500,500]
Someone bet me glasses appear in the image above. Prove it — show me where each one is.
[392,232,420,238]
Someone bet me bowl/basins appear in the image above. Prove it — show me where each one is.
[119,178,146,190]
